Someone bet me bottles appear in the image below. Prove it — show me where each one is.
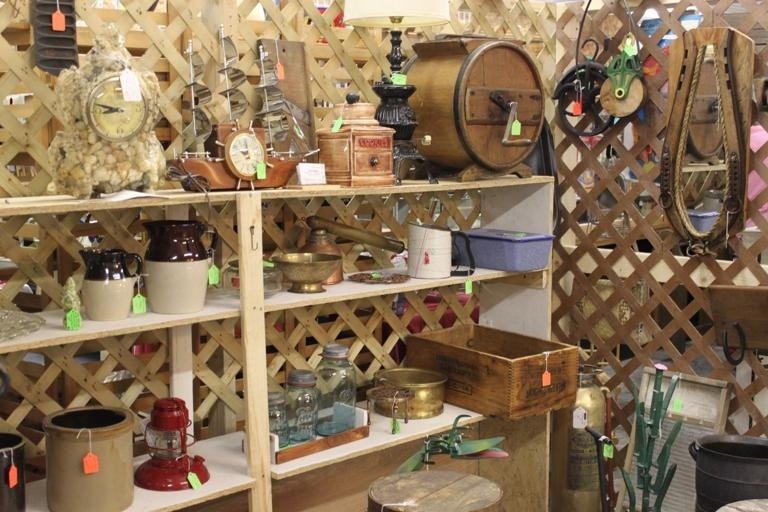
[283,367,319,442]
[313,342,355,437]
[265,392,288,448]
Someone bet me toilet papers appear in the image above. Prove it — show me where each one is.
[407,221,453,279]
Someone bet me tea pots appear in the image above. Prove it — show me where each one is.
[140,215,220,316]
[76,246,141,321]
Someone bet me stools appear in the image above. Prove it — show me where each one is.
[366,471,505,512]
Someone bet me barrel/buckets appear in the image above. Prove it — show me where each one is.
[399,33,544,171]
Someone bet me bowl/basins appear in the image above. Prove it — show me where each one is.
[373,367,449,420]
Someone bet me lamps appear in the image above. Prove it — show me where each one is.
[342,0,451,183]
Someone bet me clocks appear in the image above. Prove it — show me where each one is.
[225,131,267,181]
[86,78,150,142]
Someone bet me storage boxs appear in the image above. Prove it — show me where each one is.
[405,324,580,421]
[451,229,555,272]
[313,94,397,187]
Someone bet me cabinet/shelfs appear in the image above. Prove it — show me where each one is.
[0,173,556,512]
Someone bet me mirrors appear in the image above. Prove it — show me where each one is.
[659,26,754,260]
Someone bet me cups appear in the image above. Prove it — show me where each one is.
[404,222,476,281]
